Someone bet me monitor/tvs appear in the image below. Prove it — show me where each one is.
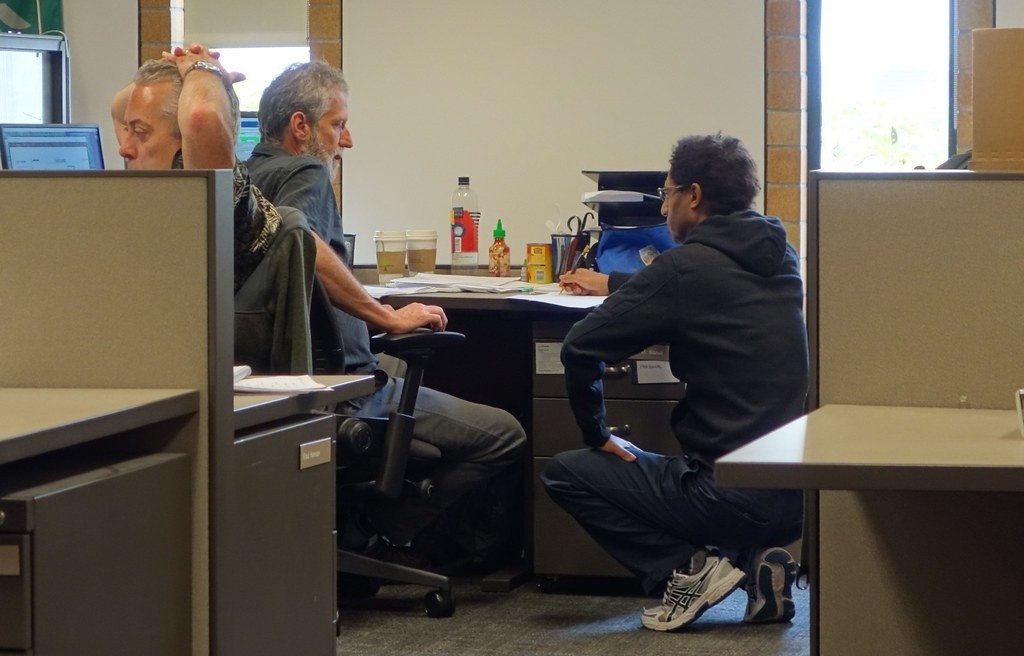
[234,111,262,162]
[0,123,105,170]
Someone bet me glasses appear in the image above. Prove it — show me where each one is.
[658,184,684,202]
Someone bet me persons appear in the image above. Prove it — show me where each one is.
[111,41,281,288]
[544,135,808,631]
[246,60,528,588]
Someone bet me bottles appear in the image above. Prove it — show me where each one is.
[520,259,528,282]
[489,218,510,277]
[450,177,479,276]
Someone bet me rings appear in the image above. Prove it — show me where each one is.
[184,49,190,53]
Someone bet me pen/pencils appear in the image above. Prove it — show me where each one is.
[564,237,578,272]
[559,245,590,295]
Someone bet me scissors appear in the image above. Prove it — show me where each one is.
[566,212,595,236]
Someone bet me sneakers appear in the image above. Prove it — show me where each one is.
[641,545,745,631]
[744,548,796,625]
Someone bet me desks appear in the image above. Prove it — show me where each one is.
[0,168,1023,656]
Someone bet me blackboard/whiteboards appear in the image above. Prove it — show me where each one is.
[339,0,768,268]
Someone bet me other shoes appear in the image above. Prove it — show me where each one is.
[346,516,426,568]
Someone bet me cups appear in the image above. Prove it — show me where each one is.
[373,230,408,287]
[406,229,438,276]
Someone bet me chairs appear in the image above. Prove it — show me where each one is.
[234,204,469,618]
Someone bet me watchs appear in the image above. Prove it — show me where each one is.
[184,59,226,89]
